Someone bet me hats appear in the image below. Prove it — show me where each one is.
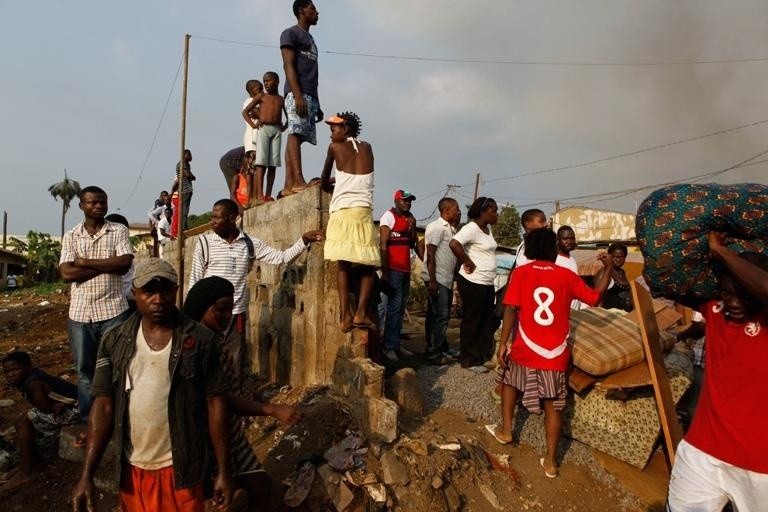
[132,257,177,288]
[325,116,344,124]
[395,190,415,200]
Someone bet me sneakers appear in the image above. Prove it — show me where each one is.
[382,344,413,361]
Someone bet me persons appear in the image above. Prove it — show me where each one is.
[57,183,227,512]
[379,189,502,372]
[150,149,195,254]
[662,236,768,512]
[219,72,289,214]
[182,197,323,510]
[486,209,632,475]
[314,113,382,332]
[280,0,323,196]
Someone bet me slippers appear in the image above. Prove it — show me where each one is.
[540,458,557,478]
[342,321,377,332]
[485,424,507,444]
